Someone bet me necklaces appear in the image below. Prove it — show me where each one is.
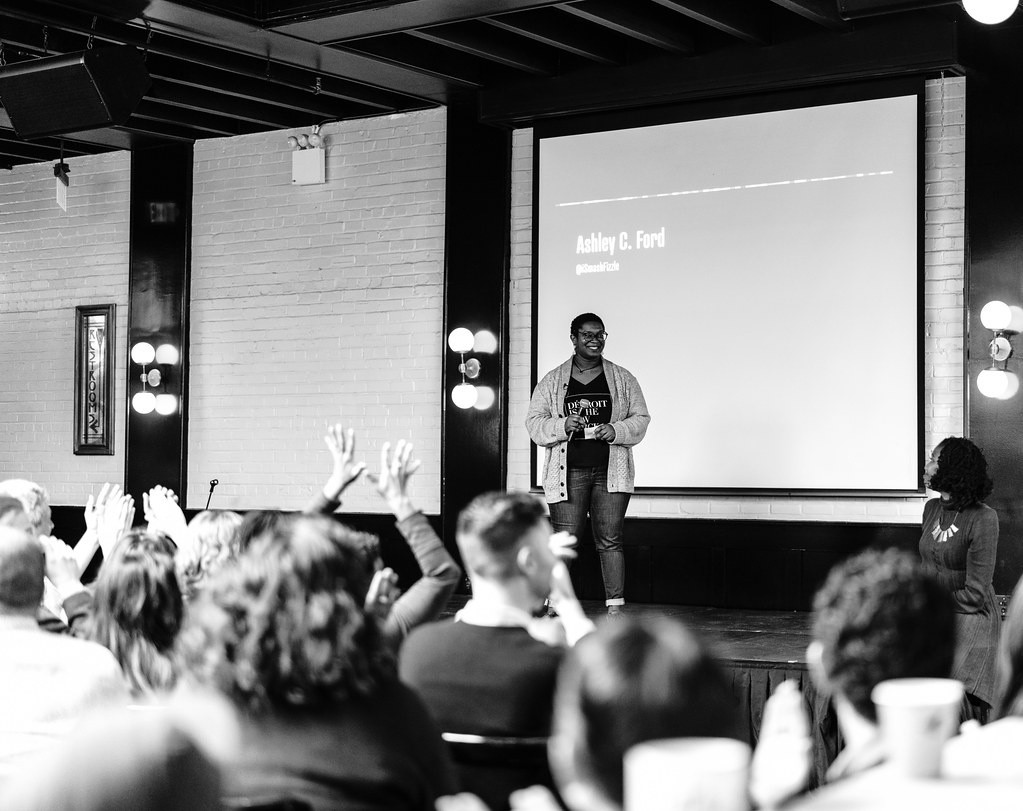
[932,506,961,543]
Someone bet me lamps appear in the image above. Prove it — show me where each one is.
[977,300,1013,398]
[471,330,498,411]
[448,327,478,409]
[287,133,326,184]
[995,306,1023,401]
[155,344,179,416]
[131,342,155,415]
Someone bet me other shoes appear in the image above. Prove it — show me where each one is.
[605,610,628,623]
[545,607,557,618]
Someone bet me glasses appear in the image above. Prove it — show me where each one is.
[577,331,608,341]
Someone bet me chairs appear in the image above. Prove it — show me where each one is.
[440,733,565,811]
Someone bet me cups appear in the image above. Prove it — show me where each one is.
[870,678,965,781]
[620,736,754,811]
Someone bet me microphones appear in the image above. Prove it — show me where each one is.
[568,398,590,443]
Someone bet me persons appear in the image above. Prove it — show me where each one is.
[919,434,1002,726]
[988,573,1023,724]
[751,546,978,811]
[525,313,651,619]
[0,423,751,811]
